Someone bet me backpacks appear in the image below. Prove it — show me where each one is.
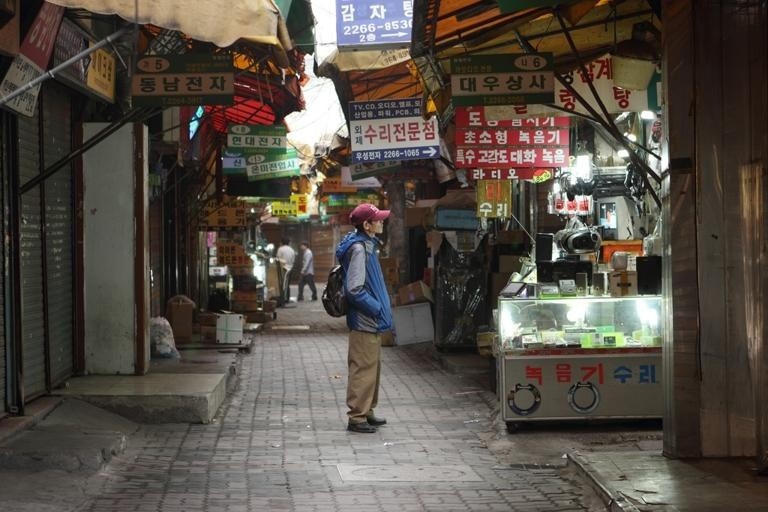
[321,240,368,318]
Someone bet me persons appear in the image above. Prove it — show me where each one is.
[297,240,317,302]
[276,238,295,303]
[335,204,395,433]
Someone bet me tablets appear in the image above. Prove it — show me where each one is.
[499,281,527,297]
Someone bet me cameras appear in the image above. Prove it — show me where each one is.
[560,284,575,294]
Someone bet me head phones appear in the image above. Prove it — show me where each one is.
[556,162,647,202]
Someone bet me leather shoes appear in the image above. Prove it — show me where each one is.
[346,416,386,433]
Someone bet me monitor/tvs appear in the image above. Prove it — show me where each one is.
[596,196,648,239]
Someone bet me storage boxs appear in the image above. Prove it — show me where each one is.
[380,254,432,351]
[406,149,437,230]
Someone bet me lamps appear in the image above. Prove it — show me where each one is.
[608,20,664,91]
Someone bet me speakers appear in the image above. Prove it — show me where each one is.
[592,272,605,297]
[636,255,662,297]
[535,232,553,262]
[575,272,587,296]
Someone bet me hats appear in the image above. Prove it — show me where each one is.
[349,203,391,226]
